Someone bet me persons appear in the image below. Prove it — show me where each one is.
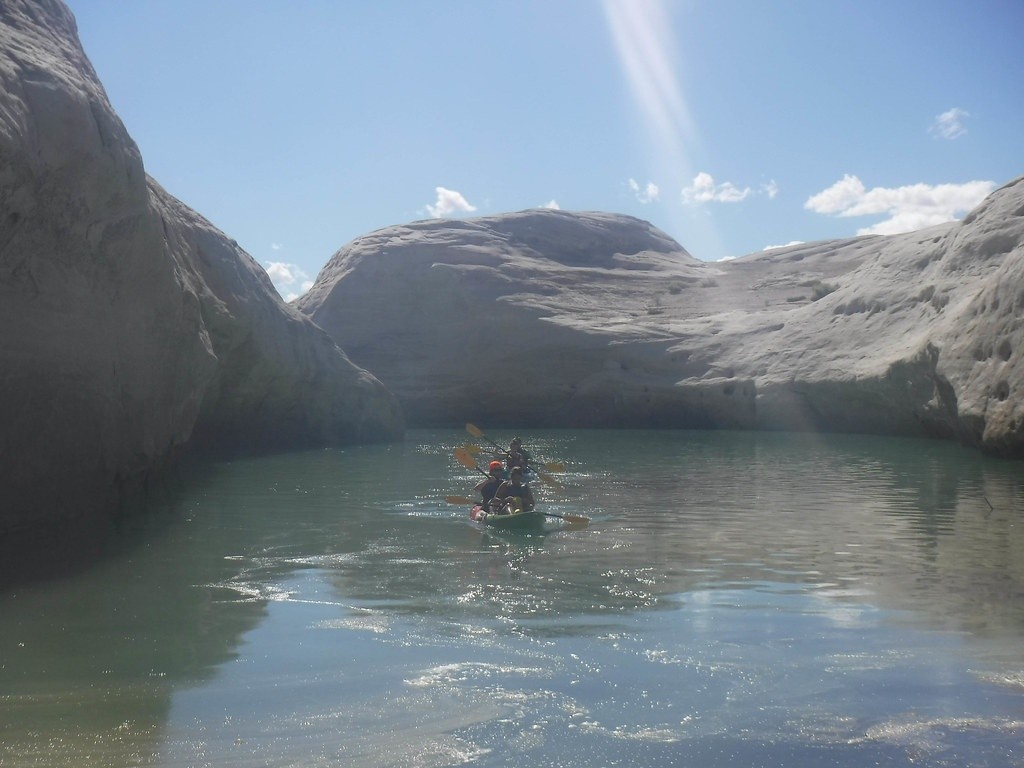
[507,435,529,470]
[479,461,510,514]
[494,466,534,512]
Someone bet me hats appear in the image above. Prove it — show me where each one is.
[489,461,500,476]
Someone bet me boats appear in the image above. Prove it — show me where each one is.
[470,503,548,531]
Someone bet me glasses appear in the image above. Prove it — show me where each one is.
[494,468,504,472]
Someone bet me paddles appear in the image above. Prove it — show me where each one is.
[452,447,491,479]
[464,443,565,473]
[443,495,590,526]
[464,422,555,483]
